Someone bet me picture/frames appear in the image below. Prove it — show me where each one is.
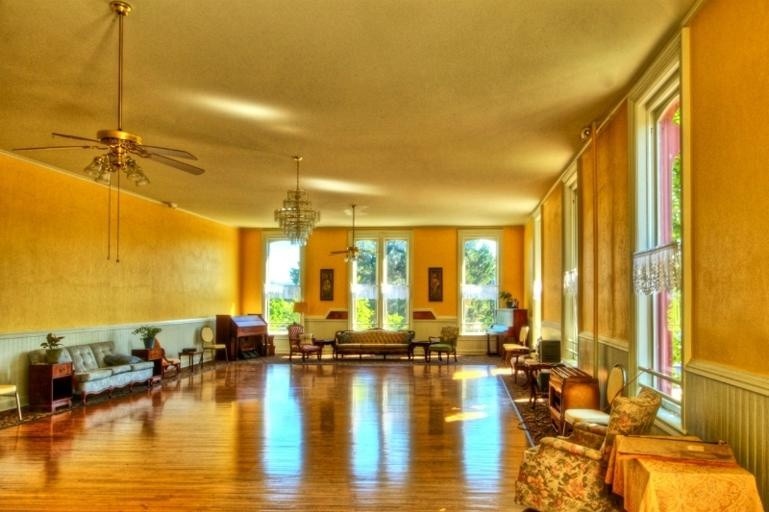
[320,269,333,302]
[428,266,443,302]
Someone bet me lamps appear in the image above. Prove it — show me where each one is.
[273,155,321,249]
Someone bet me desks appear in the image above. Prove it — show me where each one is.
[604,435,764,512]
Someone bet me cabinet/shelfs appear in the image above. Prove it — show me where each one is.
[132,347,164,388]
[214,313,269,361]
[28,362,75,413]
[485,308,527,357]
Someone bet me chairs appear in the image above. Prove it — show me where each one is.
[287,322,321,362]
[428,326,459,363]
[0,382,23,422]
[199,325,229,367]
[502,325,662,511]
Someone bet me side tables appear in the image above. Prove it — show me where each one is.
[313,337,336,361]
[178,351,203,372]
[408,339,432,363]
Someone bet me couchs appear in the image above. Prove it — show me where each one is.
[335,329,415,361]
[28,340,155,407]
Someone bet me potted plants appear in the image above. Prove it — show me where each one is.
[131,326,160,350]
[497,291,512,308]
[40,333,65,363]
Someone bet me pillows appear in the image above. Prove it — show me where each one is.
[102,353,142,366]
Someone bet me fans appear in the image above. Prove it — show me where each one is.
[330,205,370,262]
[13,3,205,187]
